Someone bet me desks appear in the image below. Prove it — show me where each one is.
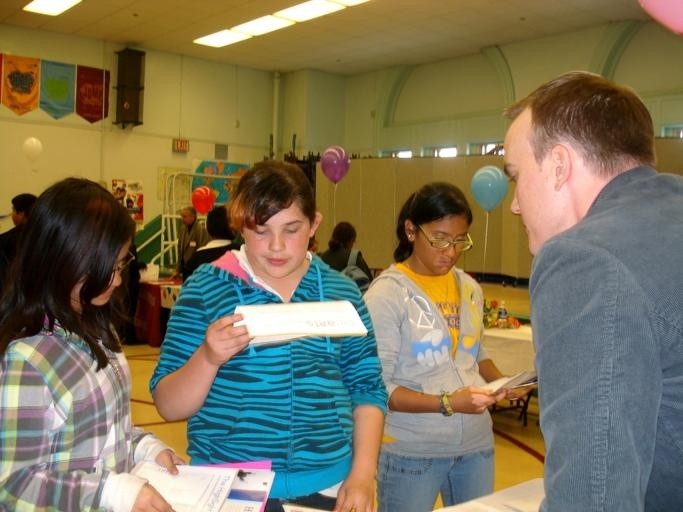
[133,277,183,347]
[481,322,538,427]
[434,478,545,512]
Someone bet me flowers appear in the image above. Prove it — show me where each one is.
[482,298,519,328]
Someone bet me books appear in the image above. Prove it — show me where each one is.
[131,457,277,511]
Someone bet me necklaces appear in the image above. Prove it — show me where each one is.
[415,266,451,308]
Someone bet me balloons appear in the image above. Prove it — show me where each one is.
[319,146,350,183]
[190,187,215,216]
[469,164,510,212]
[637,1,681,41]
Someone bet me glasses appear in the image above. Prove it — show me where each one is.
[113,248,135,275]
[419,224,474,252]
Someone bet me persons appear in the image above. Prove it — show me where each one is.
[145,159,386,512]
[501,71,682,511]
[319,221,373,294]
[169,206,206,284]
[182,207,240,281]
[0,193,37,269]
[121,240,148,344]
[361,180,532,511]
[0,177,186,511]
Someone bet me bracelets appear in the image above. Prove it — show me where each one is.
[441,392,452,417]
[438,396,447,417]
